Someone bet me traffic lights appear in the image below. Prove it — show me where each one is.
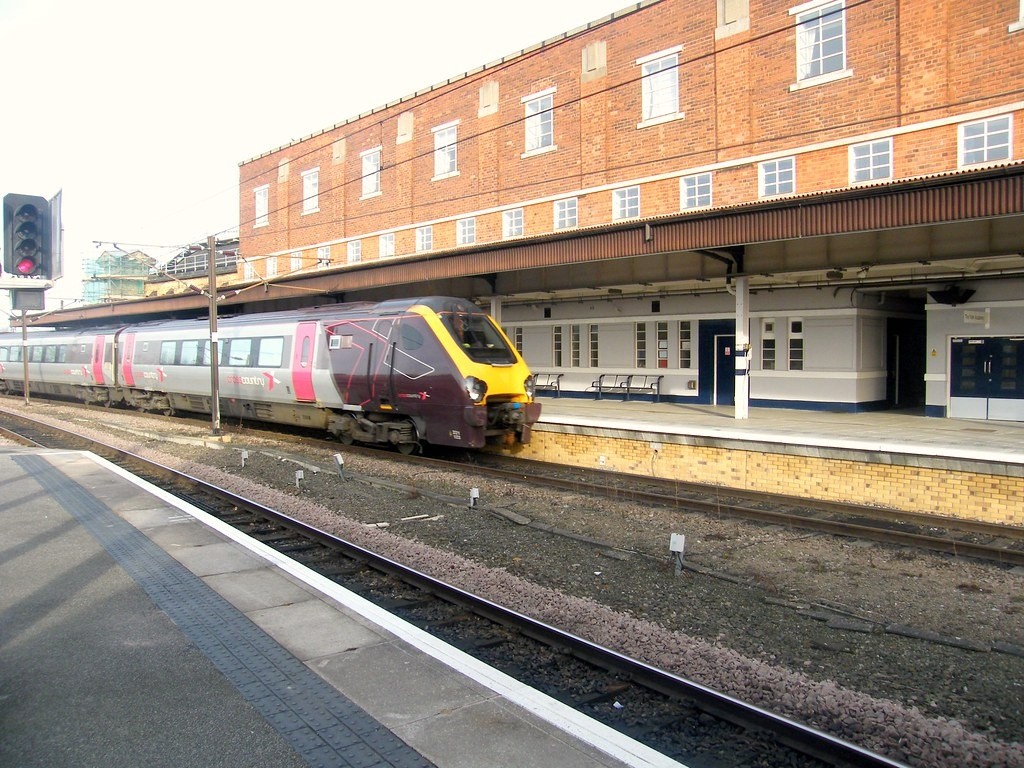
[3,193,52,281]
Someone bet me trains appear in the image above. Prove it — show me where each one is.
[0,297,542,464]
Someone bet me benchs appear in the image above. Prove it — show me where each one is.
[532,373,564,398]
[590,373,664,403]
